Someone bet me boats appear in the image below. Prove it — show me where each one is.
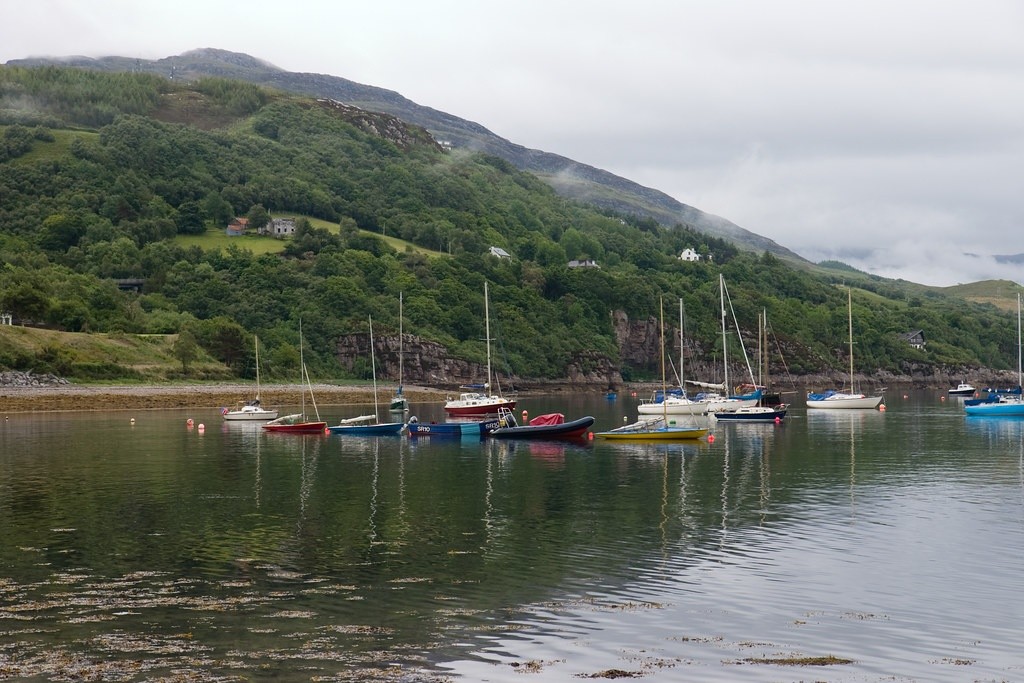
[490,411,597,438]
[948,384,977,395]
[404,416,506,433]
[445,415,503,425]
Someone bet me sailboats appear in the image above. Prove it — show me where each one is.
[221,335,280,419]
[595,298,709,439]
[389,290,410,413]
[442,279,519,416]
[327,312,407,434]
[805,286,883,409]
[637,271,800,424]
[962,290,1024,415]
[260,316,331,437]
